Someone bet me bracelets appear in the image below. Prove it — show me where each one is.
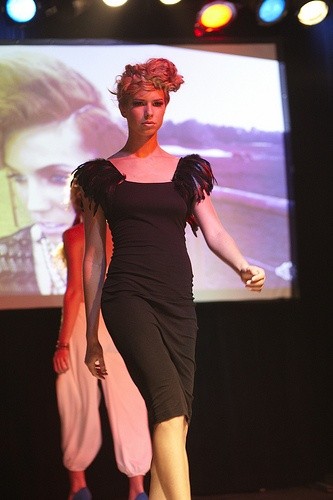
[56,340,71,350]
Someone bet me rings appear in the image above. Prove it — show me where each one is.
[96,372,99,374]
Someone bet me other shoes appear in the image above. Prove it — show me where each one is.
[135,493,148,500]
[72,488,92,500]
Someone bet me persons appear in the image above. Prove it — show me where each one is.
[69,58,267,500]
[0,53,129,294]
[54,178,153,500]
